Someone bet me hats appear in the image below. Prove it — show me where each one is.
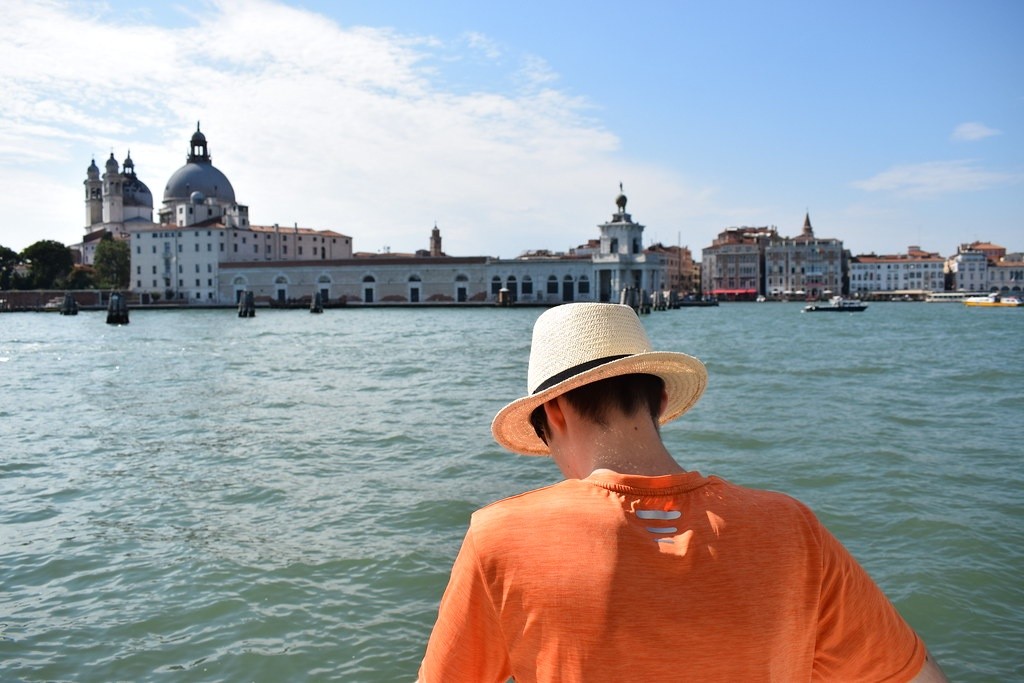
[491,302,708,456]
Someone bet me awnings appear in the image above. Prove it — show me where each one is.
[704,288,756,293]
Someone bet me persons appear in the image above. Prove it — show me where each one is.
[417,302,949,682]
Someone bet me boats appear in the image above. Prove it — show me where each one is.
[925,293,997,303]
[37,297,80,312]
[755,295,766,303]
[805,296,868,311]
[962,292,1023,307]
[891,295,914,301]
